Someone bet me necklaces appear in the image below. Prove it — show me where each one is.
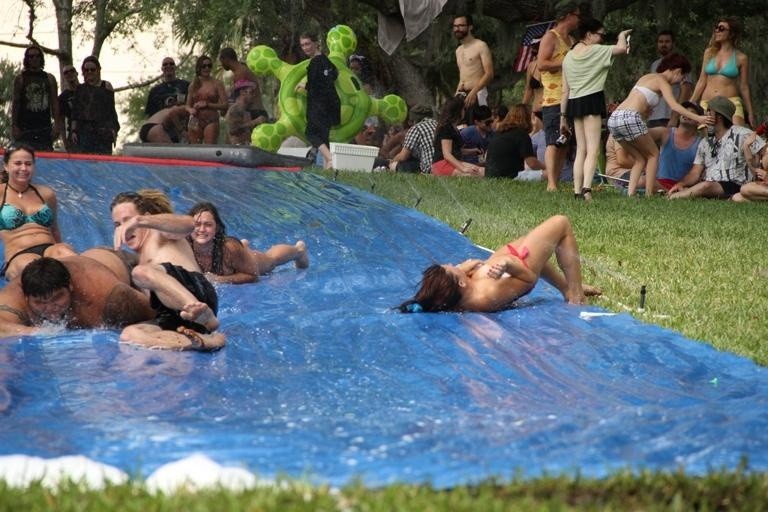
[5,182,30,200]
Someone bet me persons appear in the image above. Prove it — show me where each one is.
[0,247,159,338]
[182,200,310,283]
[61,55,120,156]
[294,31,342,169]
[138,49,270,146]
[605,17,768,202]
[0,141,76,283]
[348,32,573,181]
[537,2,633,201]
[9,45,61,153]
[388,214,603,315]
[110,187,226,353]
[451,11,494,107]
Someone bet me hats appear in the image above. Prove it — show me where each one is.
[231,80,256,92]
[681,101,704,116]
[472,105,492,120]
[707,95,736,126]
[408,102,433,120]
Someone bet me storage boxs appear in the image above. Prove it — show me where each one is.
[329,142,380,173]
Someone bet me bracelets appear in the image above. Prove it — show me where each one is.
[10,122,16,128]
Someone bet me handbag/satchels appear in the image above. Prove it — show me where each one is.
[96,127,114,144]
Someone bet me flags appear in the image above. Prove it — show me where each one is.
[514,22,557,71]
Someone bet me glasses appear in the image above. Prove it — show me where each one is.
[715,24,730,32]
[200,63,212,70]
[591,31,606,38]
[452,24,468,28]
[28,53,41,58]
[83,67,97,72]
[5,142,34,160]
[163,62,175,67]
[569,12,582,19]
[485,119,494,126]
[63,68,75,75]
